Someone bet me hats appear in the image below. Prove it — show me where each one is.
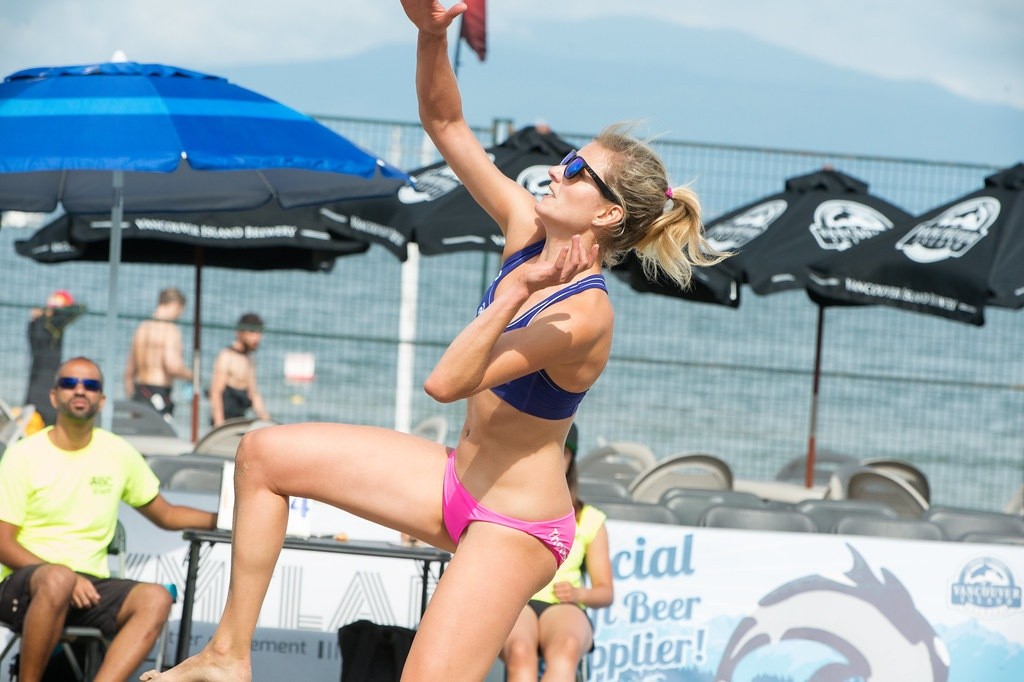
[47,290,74,308]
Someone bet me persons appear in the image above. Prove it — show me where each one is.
[139,0,728,682]
[499,423,614,682]
[23,289,83,428]
[209,313,267,426]
[123,288,193,419]
[0,357,216,682]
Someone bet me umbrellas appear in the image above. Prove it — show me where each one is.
[0,61,741,441]
[688,165,985,488]
[810,160,1024,310]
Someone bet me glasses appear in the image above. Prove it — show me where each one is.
[53,376,103,392]
[558,147,619,205]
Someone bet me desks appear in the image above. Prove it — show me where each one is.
[120,435,196,455]
[174,529,451,666]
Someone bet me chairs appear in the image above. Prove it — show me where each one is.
[0,519,178,682]
[413,418,1024,546]
[0,400,280,492]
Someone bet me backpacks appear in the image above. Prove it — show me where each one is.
[335,618,418,682]
